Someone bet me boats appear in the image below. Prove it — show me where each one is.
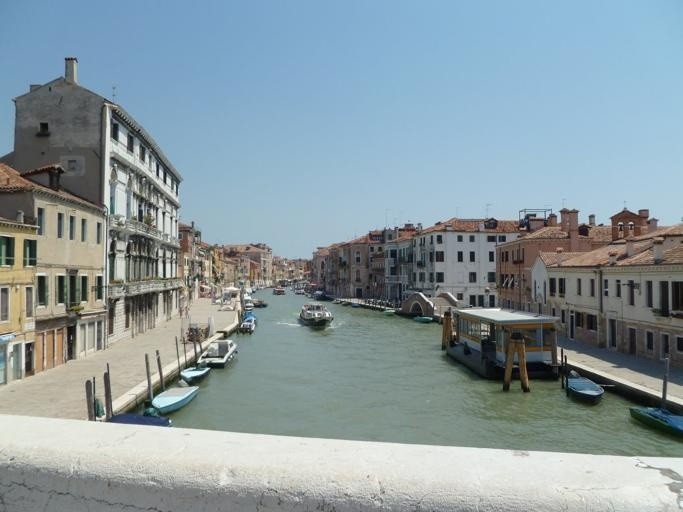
[413,314,433,322]
[273,284,285,295]
[628,405,683,438]
[151,385,200,414]
[236,284,267,334]
[300,303,334,326]
[563,369,605,405]
[446,306,561,380]
[290,281,327,300]
[180,366,212,384]
[197,339,239,368]
[106,413,172,426]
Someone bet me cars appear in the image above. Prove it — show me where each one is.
[223,293,231,302]
[367,296,401,308]
